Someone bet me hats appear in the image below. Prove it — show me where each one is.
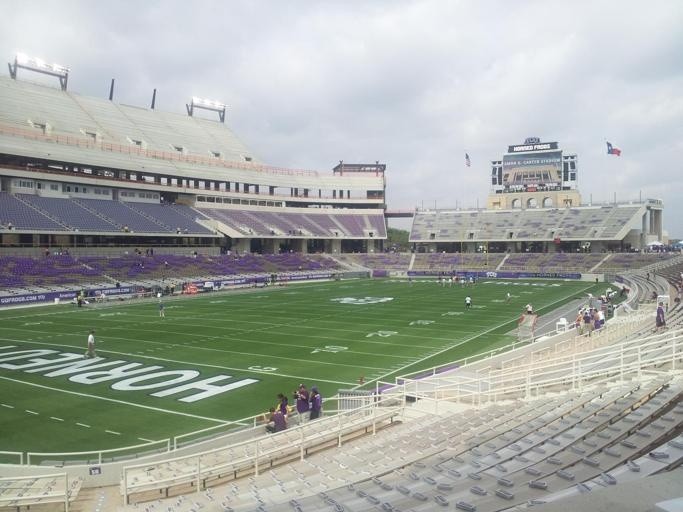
[311,388,318,392]
[298,383,305,388]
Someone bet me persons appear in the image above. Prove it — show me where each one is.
[88,331,95,352]
[160,302,164,318]
[295,384,309,421]
[264,407,286,433]
[309,387,321,420]
[278,394,288,422]
[9,221,12,230]
[45,224,339,307]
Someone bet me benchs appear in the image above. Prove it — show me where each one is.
[0,463,82,511]
[123,374,683,512]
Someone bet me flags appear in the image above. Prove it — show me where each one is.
[466,154,472,168]
[607,141,621,156]
